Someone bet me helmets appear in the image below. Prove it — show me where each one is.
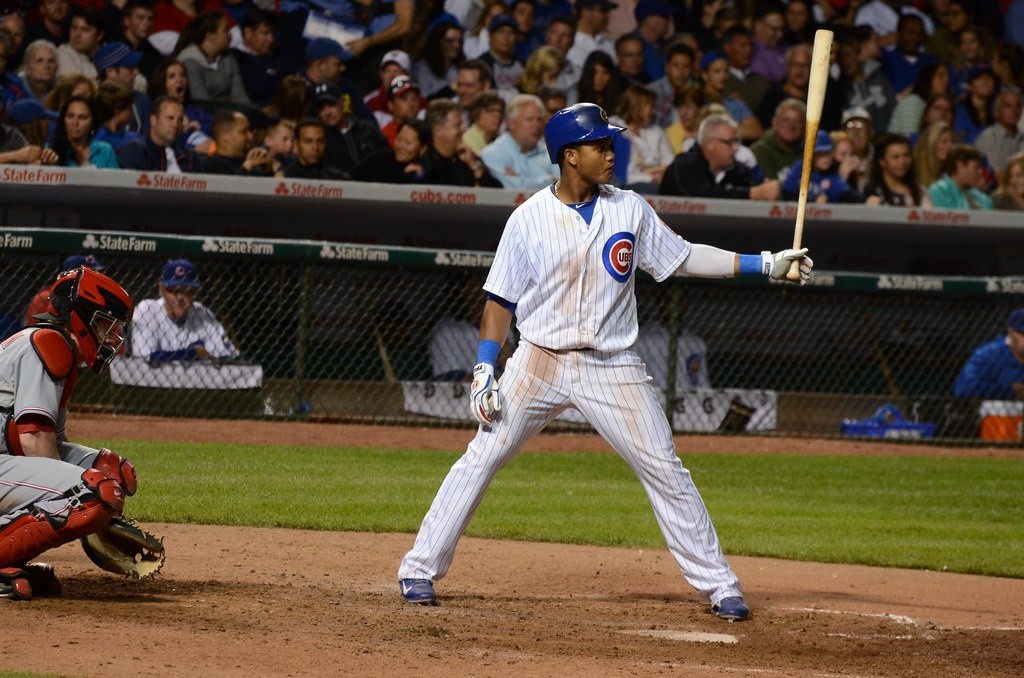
[1007,309,1024,332]
[48,263,134,376]
[541,102,628,164]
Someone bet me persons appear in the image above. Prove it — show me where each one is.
[953,309,1024,399]
[0,0,1024,223]
[0,265,138,600]
[25,255,130,413]
[397,103,813,620]
[130,258,242,368]
[428,264,709,389]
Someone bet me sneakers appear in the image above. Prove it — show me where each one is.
[399,578,435,603]
[712,597,749,619]
[0,580,13,597]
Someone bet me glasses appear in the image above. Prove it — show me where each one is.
[845,120,866,128]
[704,137,740,147]
[0,7,27,19]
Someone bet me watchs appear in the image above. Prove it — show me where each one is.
[472,157,484,173]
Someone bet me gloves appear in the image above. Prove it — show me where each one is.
[469,362,502,427]
[761,247,814,286]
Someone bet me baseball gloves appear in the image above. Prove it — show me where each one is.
[79,514,165,580]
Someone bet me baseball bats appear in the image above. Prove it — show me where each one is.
[786,29,836,281]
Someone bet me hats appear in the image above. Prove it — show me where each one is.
[966,60,991,80]
[488,12,518,32]
[840,106,872,126]
[304,37,354,62]
[814,128,833,152]
[575,0,618,13]
[160,257,201,288]
[423,11,463,41]
[93,40,142,72]
[701,48,731,71]
[380,49,411,70]
[9,99,60,125]
[309,79,340,108]
[386,74,421,97]
[62,254,105,272]
